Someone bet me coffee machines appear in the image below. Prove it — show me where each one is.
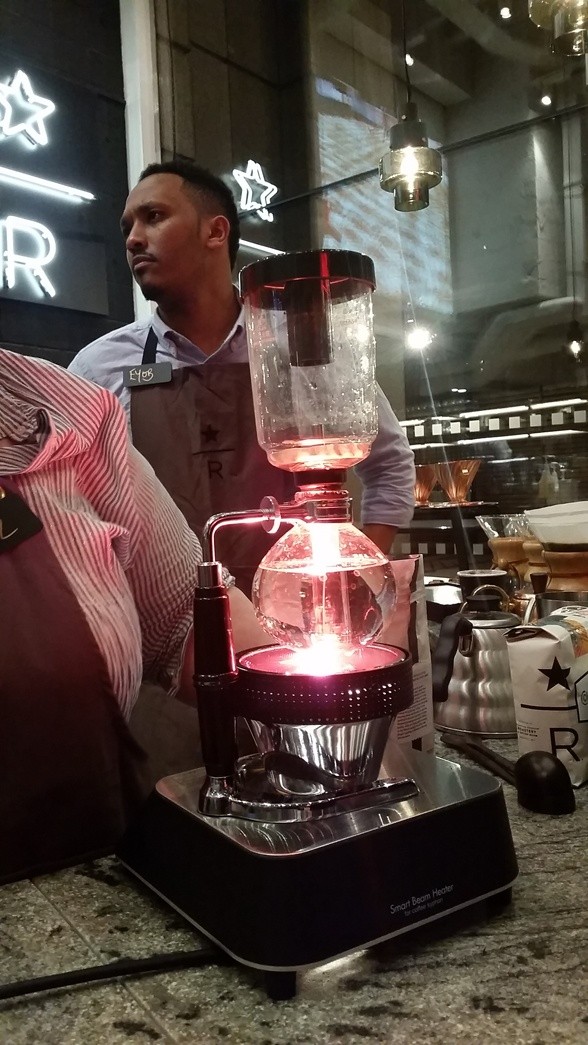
[88,202,522,1008]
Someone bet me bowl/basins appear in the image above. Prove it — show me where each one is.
[434,457,483,505]
[412,459,441,510]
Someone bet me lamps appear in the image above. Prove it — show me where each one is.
[527,0,588,57]
[373,0,442,212]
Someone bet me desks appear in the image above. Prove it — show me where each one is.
[0,729,588,1045]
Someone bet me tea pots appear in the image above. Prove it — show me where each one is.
[430,581,550,741]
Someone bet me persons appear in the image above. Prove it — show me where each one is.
[61,159,421,812]
[0,318,290,922]
